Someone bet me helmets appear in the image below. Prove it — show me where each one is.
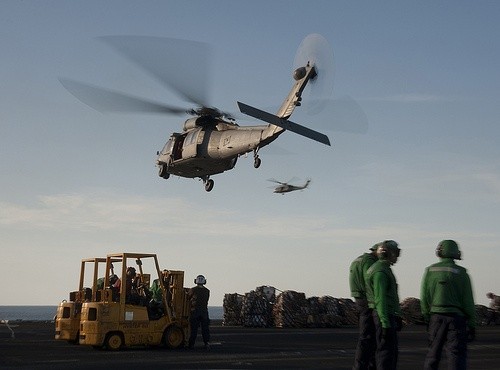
[377,240,400,263]
[437,240,460,258]
[110,275,118,284]
[370,242,383,252]
[194,275,206,285]
[126,267,136,278]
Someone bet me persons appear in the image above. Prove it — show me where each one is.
[482,292,500,327]
[137,281,172,319]
[185,275,210,350]
[115,267,139,294]
[420,240,478,370]
[96,274,117,289]
[150,270,169,300]
[349,240,402,370]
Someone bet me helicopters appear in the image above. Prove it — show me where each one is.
[64,33,332,192]
[263,177,311,195]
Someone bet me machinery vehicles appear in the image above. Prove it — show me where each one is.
[53,252,194,350]
